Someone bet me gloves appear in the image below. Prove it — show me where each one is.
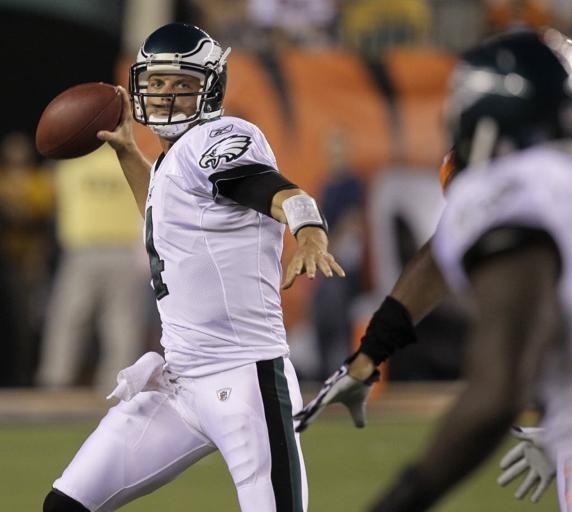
[497,425,556,503]
[293,359,380,432]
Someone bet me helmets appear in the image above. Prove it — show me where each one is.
[444,24,571,135]
[128,23,231,119]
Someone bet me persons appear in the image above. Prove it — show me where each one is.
[0,142,152,388]
[43,24,347,512]
[293,32,572,512]
[119,0,570,386]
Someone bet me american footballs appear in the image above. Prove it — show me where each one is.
[34,81,122,159]
